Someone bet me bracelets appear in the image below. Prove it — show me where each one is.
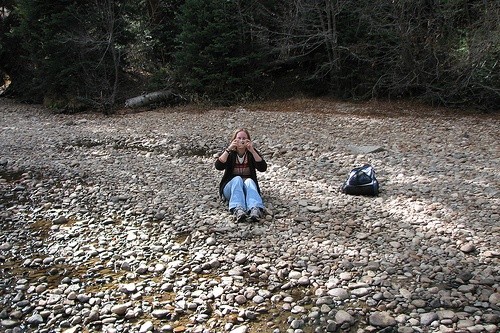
[225,149,230,154]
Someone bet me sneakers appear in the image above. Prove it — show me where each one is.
[232,210,245,223]
[249,207,262,222]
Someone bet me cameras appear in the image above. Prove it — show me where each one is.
[237,140,247,148]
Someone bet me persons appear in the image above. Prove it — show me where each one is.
[214,128,268,224]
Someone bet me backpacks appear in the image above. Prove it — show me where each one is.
[344,166,379,196]
